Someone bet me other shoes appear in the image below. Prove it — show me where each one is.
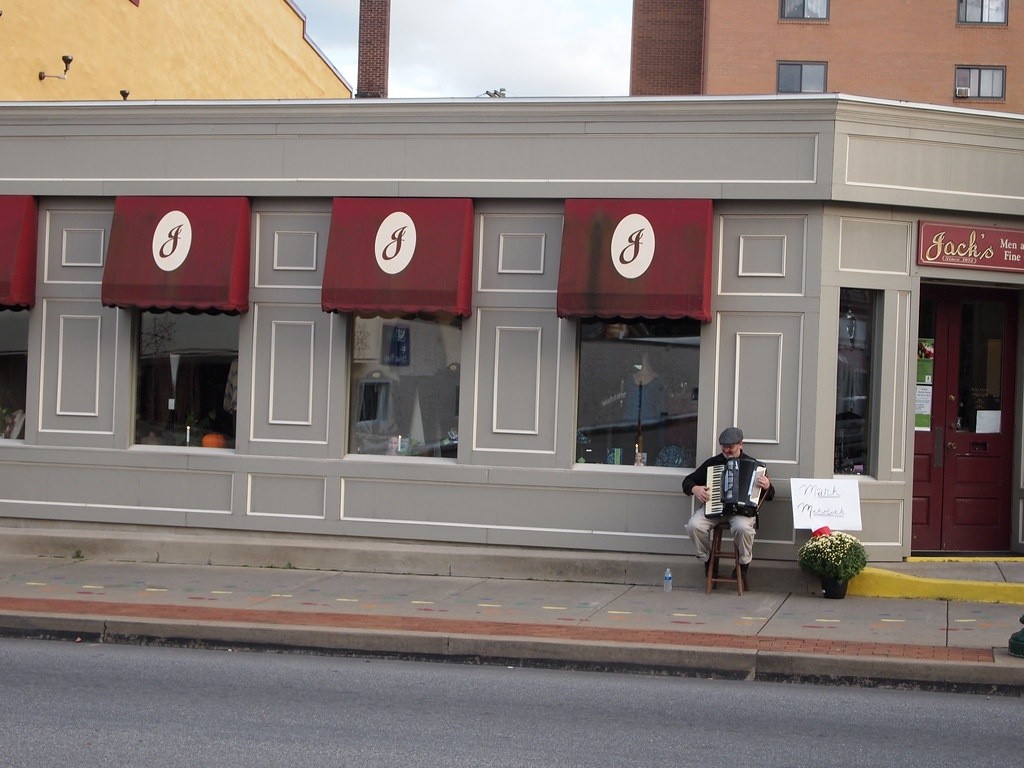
[732,563,749,579]
[704,551,719,579]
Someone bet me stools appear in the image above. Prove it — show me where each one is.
[705,523,749,596]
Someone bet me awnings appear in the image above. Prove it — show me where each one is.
[1,192,40,309]
[556,196,715,322]
[102,195,253,318]
[321,195,475,326]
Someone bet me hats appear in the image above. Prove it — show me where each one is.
[719,427,744,445]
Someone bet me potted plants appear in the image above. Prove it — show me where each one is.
[796,525,867,601]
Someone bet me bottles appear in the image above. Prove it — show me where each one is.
[664,568,672,592]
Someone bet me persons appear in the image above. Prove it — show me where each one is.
[619,351,669,439]
[838,343,869,420]
[146,350,205,446]
[683,426,776,581]
